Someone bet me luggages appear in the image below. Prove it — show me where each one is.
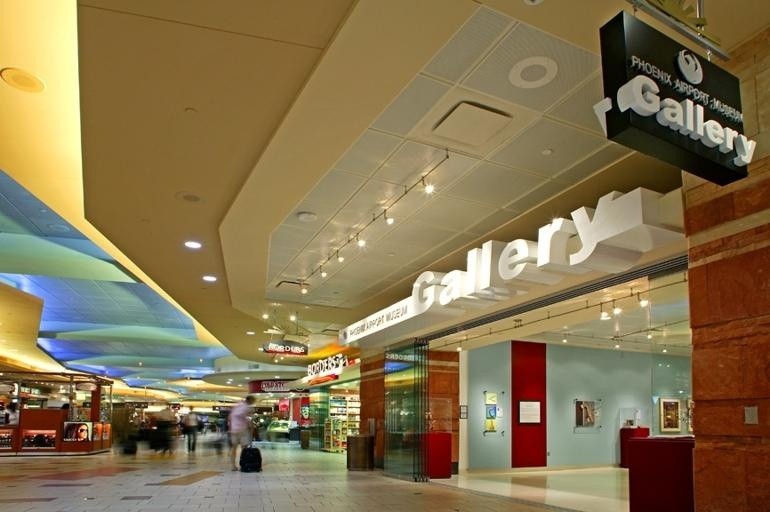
[240,421,262,472]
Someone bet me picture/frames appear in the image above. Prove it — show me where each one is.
[658,398,683,433]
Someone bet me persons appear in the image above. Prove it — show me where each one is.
[5,402,18,424]
[111,396,279,471]
[63,423,89,441]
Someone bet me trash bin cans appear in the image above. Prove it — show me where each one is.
[301,430,309,449]
[347,434,375,471]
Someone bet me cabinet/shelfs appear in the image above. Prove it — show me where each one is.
[321,390,362,453]
